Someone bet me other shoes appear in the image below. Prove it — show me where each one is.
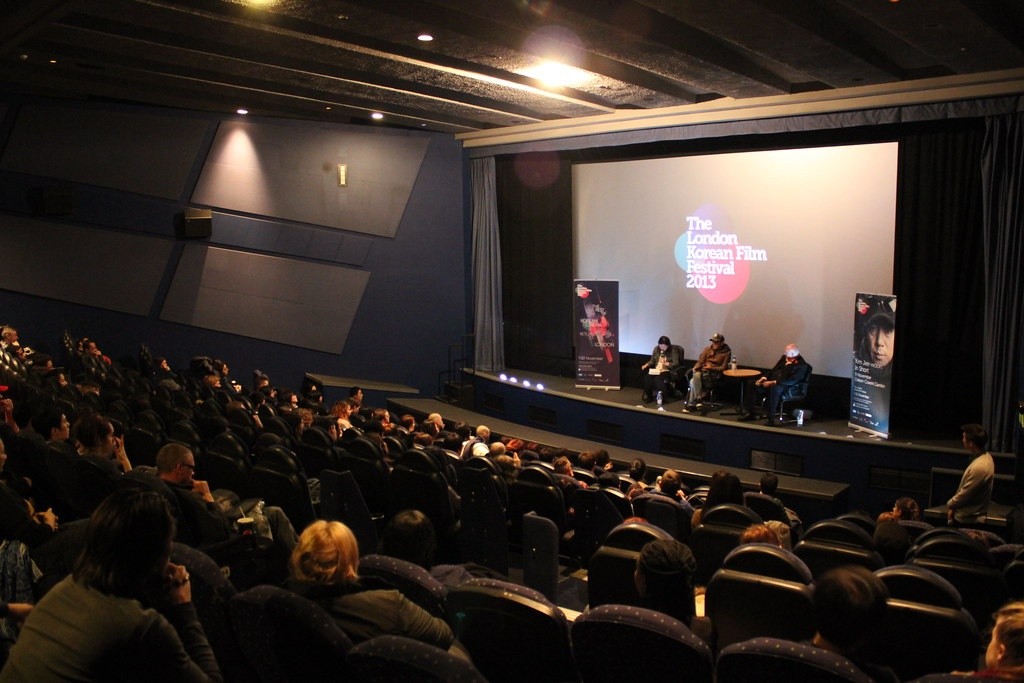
[682,406,692,413]
[644,395,653,404]
[767,414,784,427]
[695,399,703,407]
[737,413,757,422]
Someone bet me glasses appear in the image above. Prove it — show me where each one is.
[181,463,197,476]
[861,296,896,317]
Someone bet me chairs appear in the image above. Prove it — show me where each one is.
[642,345,685,402]
[755,364,811,424]
[686,368,723,408]
[0,360,1024,683]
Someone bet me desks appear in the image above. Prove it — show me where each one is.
[306,372,418,408]
[720,369,762,416]
[389,398,850,500]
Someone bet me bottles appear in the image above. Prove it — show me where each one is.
[657,391,662,408]
[732,355,736,370]
[797,410,803,427]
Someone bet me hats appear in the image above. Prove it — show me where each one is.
[860,311,896,327]
[471,442,490,457]
[657,336,670,345]
[709,333,725,342]
[429,412,445,428]
[783,344,800,358]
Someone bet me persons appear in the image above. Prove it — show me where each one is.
[688,333,732,411]
[737,344,807,426]
[851,293,896,434]
[0,324,1024,683]
[642,336,680,404]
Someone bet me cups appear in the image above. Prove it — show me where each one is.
[238,517,253,534]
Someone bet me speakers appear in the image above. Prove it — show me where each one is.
[183,209,212,238]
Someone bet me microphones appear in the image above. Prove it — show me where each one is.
[660,350,664,357]
